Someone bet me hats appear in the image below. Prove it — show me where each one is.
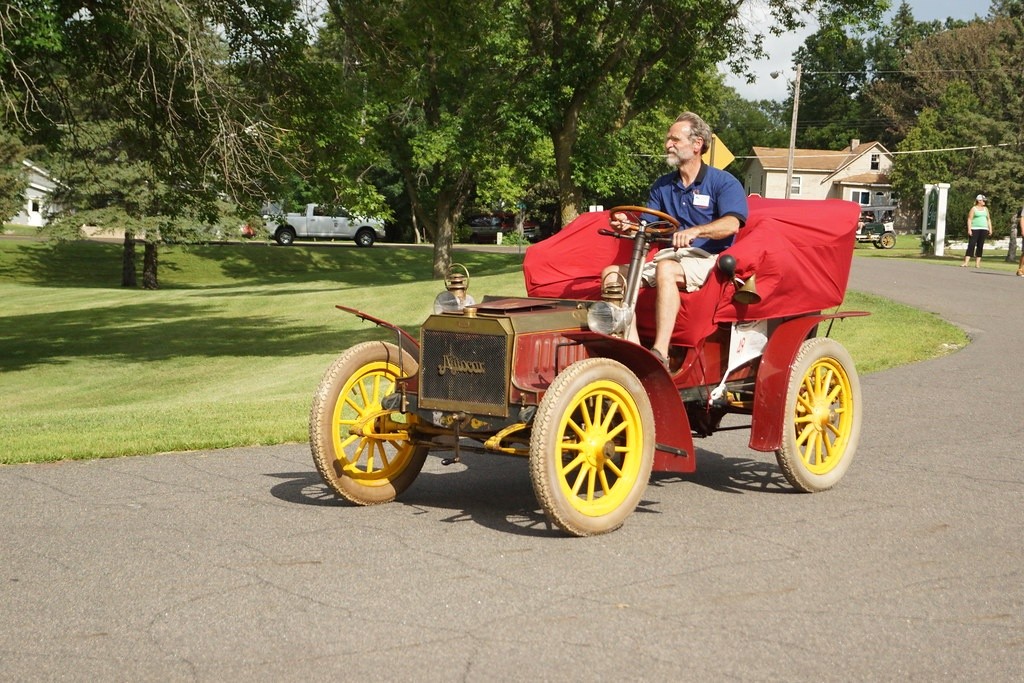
[975,194,987,201]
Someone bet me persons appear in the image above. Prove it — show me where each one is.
[596,111,749,376]
[1016,205,1024,278]
[959,194,993,268]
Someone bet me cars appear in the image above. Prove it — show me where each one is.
[457,212,552,244]
[309,191,863,538]
[856,219,896,249]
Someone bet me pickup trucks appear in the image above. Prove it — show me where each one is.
[261,205,385,247]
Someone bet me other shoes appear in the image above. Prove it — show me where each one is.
[960,264,968,267]
[651,348,671,372]
[1016,269,1024,277]
[975,264,980,268]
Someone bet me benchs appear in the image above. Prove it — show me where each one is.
[522,196,862,350]
[874,221,893,234]
